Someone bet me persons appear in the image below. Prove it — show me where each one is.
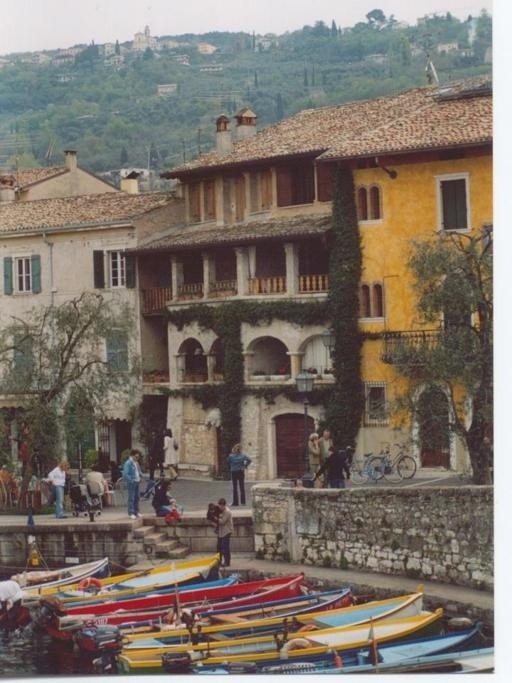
[151,481,180,516]
[149,430,179,480]
[226,444,251,506]
[308,430,349,488]
[211,499,232,566]
[47,463,68,518]
[123,450,142,519]
[86,466,110,505]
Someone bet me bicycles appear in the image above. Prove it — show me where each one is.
[313,443,417,484]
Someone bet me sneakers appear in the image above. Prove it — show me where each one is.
[130,513,143,519]
[221,563,229,566]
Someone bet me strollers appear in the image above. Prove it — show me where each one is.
[69,479,103,517]
[143,478,162,501]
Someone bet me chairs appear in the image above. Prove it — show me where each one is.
[101,476,127,507]
